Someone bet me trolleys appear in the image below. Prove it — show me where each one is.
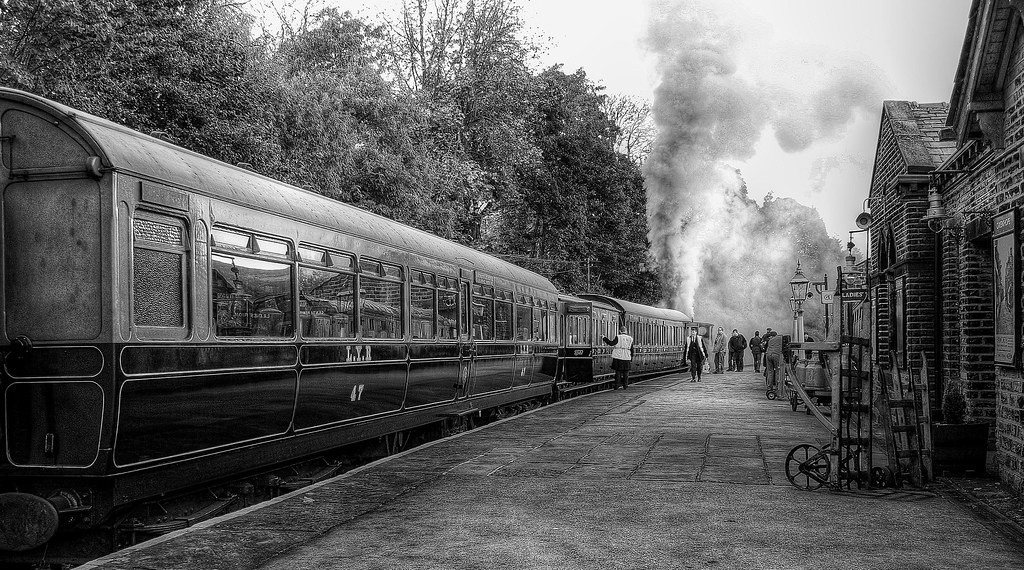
[886,347,935,488]
[779,265,874,494]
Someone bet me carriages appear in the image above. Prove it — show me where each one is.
[869,349,924,491]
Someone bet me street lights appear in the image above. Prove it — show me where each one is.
[789,292,799,356]
[789,257,811,363]
[807,273,829,341]
[846,230,873,302]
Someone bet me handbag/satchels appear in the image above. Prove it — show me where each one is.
[702,358,712,374]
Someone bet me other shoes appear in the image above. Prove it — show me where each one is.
[698,378,701,382]
[712,371,719,374]
[731,367,735,372]
[726,368,732,371]
[739,369,743,372]
[691,379,696,383]
[735,369,740,372]
[719,370,724,374]
[754,369,761,373]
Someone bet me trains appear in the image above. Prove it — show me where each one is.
[0,84,714,548]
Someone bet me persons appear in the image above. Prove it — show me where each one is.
[683,328,709,382]
[749,330,763,372]
[731,329,747,372]
[600,326,634,390]
[726,332,737,371]
[804,331,815,360]
[762,328,792,391]
[711,326,728,374]
